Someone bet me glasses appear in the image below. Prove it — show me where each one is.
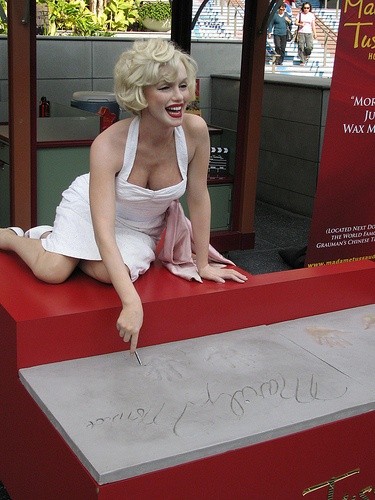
[280,9,285,11]
[303,7,310,9]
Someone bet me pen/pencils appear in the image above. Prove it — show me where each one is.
[128,338,142,365]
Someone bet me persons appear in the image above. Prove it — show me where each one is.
[268,3,291,64]
[271,0,293,42]
[0,40,247,358]
[294,2,317,64]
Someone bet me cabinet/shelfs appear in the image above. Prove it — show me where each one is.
[0,98,102,228]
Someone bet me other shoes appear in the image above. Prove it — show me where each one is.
[0,227,24,238]
[25,225,53,240]
[276,62,283,65]
[300,63,305,66]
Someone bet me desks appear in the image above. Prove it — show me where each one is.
[179,183,233,233]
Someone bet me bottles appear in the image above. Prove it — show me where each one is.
[39,96,51,118]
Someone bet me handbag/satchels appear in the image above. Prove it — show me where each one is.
[286,30,293,41]
[292,30,298,43]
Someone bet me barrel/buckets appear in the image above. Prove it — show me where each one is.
[70,91,122,133]
